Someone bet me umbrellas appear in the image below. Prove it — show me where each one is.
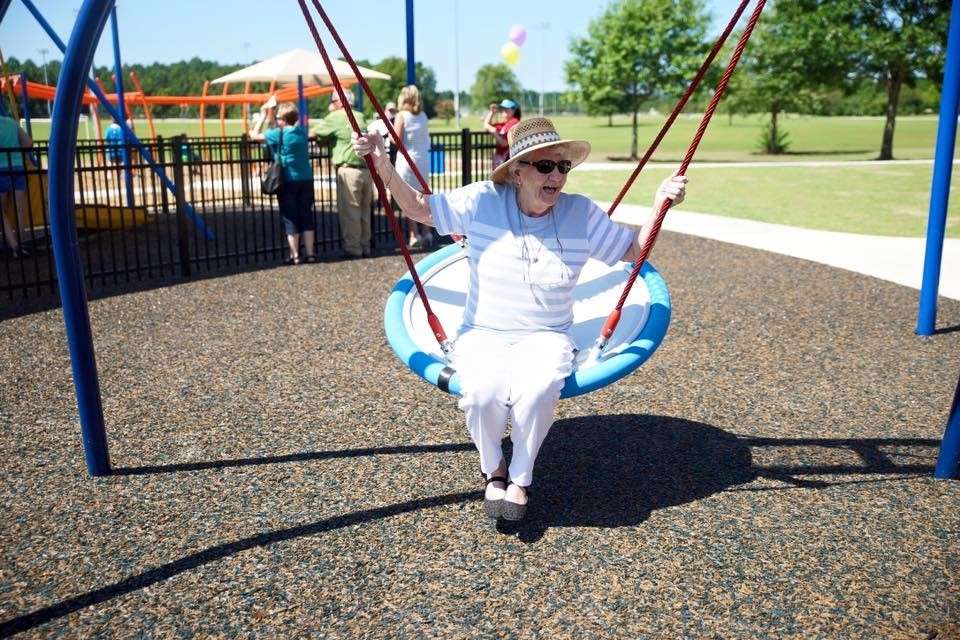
[208,49,392,136]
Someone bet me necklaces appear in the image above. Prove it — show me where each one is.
[517,200,551,265]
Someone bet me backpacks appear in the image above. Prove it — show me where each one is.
[260,160,281,195]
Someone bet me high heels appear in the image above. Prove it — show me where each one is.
[407,233,433,251]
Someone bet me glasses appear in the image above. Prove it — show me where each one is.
[331,99,338,104]
[274,113,282,120]
[516,160,573,175]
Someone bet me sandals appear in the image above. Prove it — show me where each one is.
[281,255,318,266]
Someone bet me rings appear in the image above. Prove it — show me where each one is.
[679,191,683,198]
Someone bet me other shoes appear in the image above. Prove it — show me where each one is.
[482,467,531,521]
[334,246,371,258]
[6,243,34,259]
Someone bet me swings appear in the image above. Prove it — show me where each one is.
[300,0,766,399]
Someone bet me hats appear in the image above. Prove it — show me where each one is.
[490,117,591,185]
[498,99,519,111]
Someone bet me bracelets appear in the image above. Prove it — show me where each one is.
[385,164,395,188]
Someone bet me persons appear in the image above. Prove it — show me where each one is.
[0,99,35,259]
[353,117,690,523]
[104,110,138,177]
[482,98,522,172]
[377,100,398,169]
[179,133,201,176]
[387,84,434,251]
[251,100,323,266]
[306,89,374,260]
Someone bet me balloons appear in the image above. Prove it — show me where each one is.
[499,44,522,65]
[507,26,527,46]
